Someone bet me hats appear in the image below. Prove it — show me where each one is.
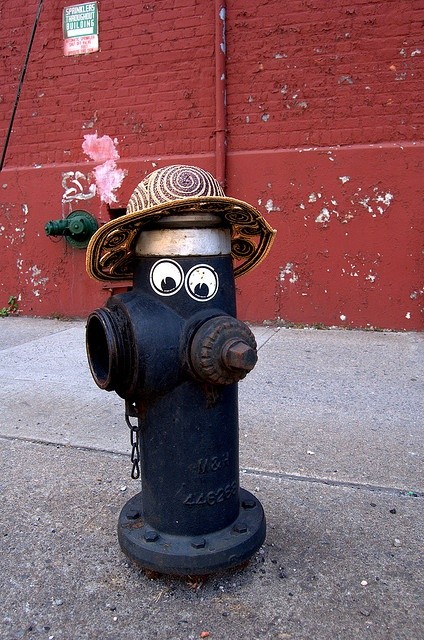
[85,162,278,285]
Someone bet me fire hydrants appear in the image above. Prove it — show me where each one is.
[86,210,266,576]
[45,209,98,248]
